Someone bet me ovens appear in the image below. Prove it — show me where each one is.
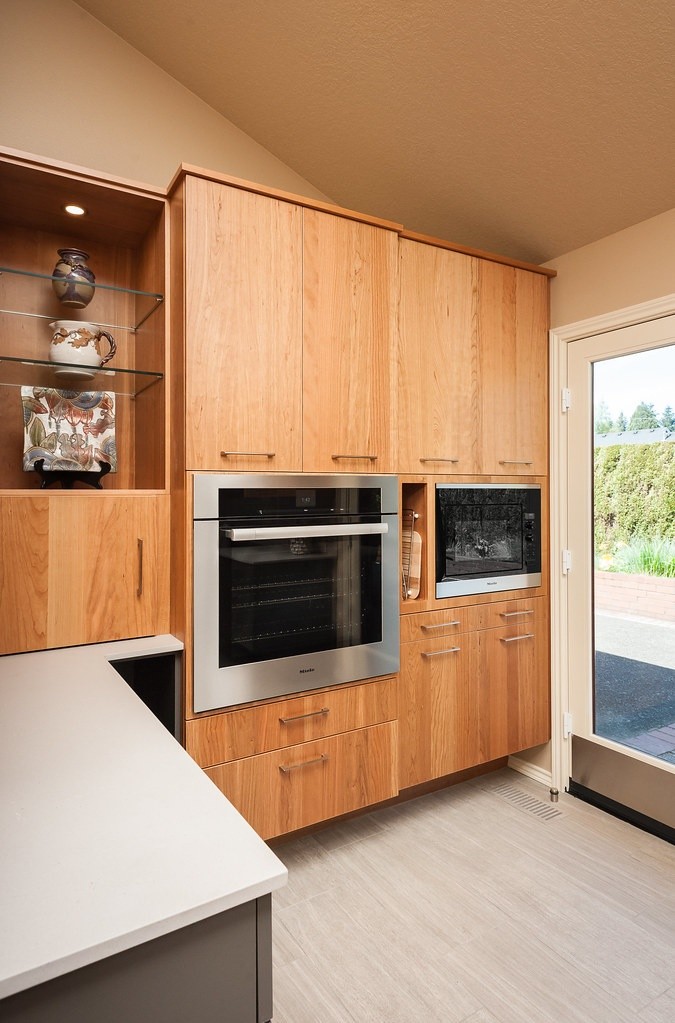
[191,473,401,715]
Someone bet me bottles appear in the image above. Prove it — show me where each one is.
[52,248,96,309]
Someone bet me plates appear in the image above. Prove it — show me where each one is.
[20,386,117,473]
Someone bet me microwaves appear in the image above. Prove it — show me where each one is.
[435,484,542,598]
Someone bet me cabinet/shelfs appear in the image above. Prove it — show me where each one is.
[0,145,557,1023]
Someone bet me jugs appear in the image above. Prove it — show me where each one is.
[48,320,117,381]
